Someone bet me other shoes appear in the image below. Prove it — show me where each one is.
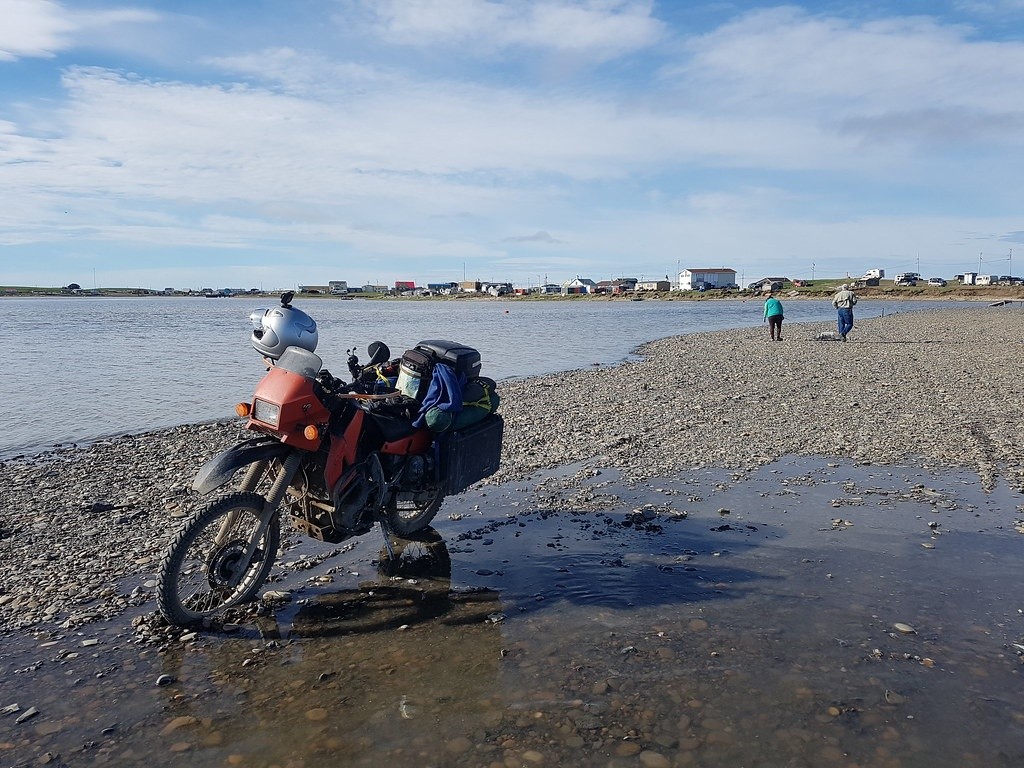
[777,338,782,341]
[771,338,774,341]
[840,333,846,342]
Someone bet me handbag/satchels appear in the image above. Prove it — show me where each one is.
[393,344,437,401]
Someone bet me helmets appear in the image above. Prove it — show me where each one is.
[250,306,319,360]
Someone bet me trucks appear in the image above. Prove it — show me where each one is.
[331,290,348,295]
[904,272,923,281]
[863,268,884,280]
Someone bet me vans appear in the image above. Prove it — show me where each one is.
[896,275,904,279]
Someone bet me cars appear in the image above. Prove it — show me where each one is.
[727,283,738,289]
[992,277,1024,286]
[928,278,947,287]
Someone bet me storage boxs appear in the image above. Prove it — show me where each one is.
[437,415,504,497]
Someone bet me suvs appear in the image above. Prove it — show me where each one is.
[748,282,759,289]
[895,278,916,286]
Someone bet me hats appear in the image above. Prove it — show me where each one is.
[842,284,848,289]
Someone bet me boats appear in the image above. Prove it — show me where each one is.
[630,298,644,301]
[341,297,353,300]
[205,292,220,297]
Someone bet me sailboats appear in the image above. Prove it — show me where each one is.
[81,267,106,297]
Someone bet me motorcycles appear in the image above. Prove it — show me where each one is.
[157,338,505,626]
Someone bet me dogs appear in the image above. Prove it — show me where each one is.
[814,331,836,340]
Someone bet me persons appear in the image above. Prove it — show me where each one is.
[763,295,784,341]
[832,284,857,342]
